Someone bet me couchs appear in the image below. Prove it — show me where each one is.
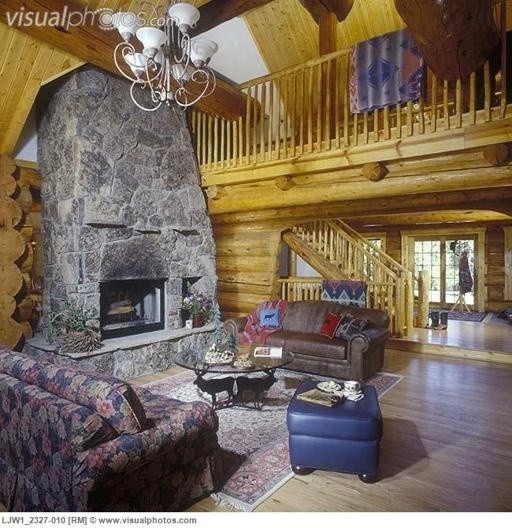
[221,300,390,383]
[0,346,219,512]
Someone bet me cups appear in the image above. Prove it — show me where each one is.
[344,381,360,391]
[186,320,193,330]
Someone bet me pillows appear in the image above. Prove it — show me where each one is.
[259,307,280,328]
[319,311,368,342]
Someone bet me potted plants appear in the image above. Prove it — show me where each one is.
[37,295,104,351]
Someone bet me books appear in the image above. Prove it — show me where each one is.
[296,388,341,408]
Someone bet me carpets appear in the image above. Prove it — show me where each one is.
[412,307,487,322]
[131,368,405,512]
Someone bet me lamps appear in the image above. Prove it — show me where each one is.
[112,1,219,118]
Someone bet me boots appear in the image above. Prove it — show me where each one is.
[425,311,440,329]
[433,313,448,330]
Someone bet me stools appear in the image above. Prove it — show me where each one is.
[287,378,384,484]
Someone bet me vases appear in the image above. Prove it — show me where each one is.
[193,313,203,327]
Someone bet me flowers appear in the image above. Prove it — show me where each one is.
[182,281,217,316]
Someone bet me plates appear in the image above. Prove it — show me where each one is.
[317,382,341,393]
